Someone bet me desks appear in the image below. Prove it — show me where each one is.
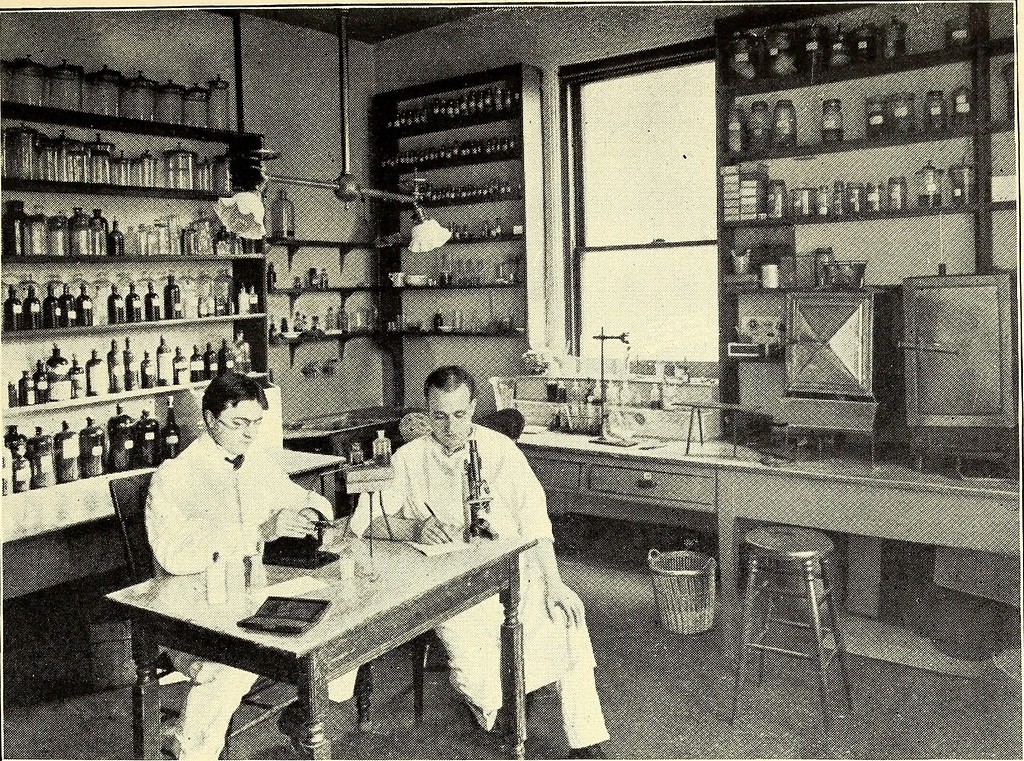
[2,449,345,600]
[104,512,539,761]
[518,426,723,621]
[716,448,1021,619]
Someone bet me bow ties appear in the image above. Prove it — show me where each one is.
[225,454,245,470]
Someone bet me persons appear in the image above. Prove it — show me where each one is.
[350,364,611,760]
[143,373,360,760]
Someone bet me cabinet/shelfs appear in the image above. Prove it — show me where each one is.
[0,6,1018,526]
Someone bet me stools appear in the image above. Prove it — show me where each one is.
[728,525,854,734]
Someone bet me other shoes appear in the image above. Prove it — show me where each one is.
[471,710,506,746]
[276,703,310,758]
[570,745,606,759]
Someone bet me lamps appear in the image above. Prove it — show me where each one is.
[212,9,455,253]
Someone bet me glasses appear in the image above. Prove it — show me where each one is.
[216,418,261,429]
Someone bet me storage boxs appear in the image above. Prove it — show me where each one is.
[739,187,767,196]
[722,199,740,206]
[723,214,740,221]
[932,545,1020,609]
[726,341,776,358]
[723,183,739,192]
[740,180,768,188]
[723,191,739,199]
[741,214,758,219]
[740,196,765,204]
[719,165,741,175]
[723,175,740,183]
[739,162,767,174]
[898,271,1017,432]
[741,205,766,213]
[844,533,893,618]
[724,207,740,214]
[740,172,768,180]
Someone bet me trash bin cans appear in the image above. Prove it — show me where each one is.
[648,547,717,634]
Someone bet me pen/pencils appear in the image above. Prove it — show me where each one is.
[425,505,452,542]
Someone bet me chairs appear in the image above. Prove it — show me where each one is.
[411,631,532,727]
[109,470,304,761]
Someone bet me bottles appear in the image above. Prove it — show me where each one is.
[542,359,692,415]
[1,193,398,499]
[714,18,981,291]
[378,83,520,336]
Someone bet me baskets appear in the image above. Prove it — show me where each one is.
[647,547,716,634]
